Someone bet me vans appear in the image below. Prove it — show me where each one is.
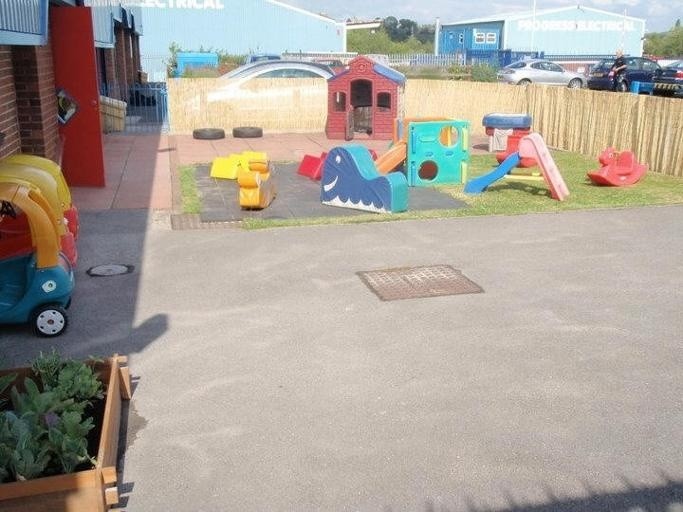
[587,57,661,92]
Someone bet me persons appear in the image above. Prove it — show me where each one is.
[610,47,628,92]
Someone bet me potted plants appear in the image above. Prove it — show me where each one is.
[0,345,131,512]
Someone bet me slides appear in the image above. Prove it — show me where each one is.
[464,152,521,193]
[373,144,409,173]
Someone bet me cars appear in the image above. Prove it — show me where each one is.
[496,59,587,88]
[217,59,344,79]
[652,61,683,95]
